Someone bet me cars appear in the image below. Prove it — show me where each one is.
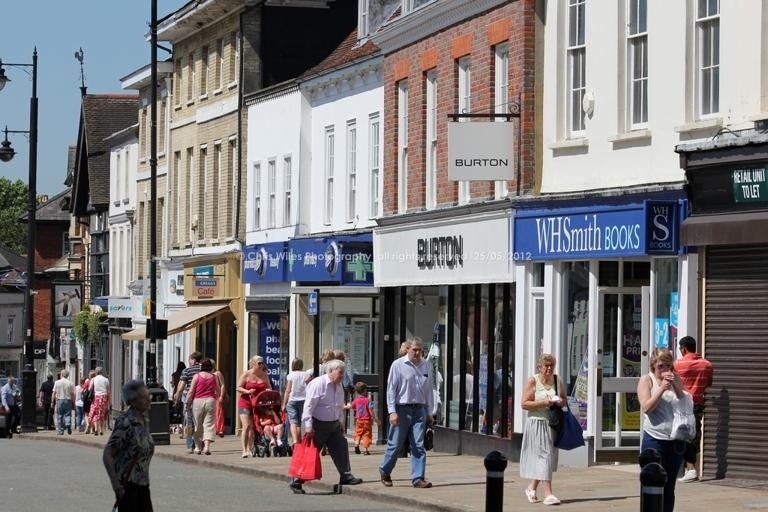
[0,377,54,438]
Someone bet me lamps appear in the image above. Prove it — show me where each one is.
[406,287,428,306]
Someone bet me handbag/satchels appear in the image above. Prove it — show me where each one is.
[183,425,192,437]
[219,390,230,402]
[547,402,564,431]
[423,426,434,451]
[288,442,322,480]
[552,410,585,450]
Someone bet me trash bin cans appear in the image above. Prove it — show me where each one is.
[146,388,170,445]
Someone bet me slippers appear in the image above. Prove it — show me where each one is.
[543,494,561,505]
[525,487,540,503]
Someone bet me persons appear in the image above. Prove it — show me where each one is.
[102,378,159,511]
[672,335,713,483]
[635,347,698,511]
[519,352,567,506]
[286,358,364,495]
[447,347,512,436]
[376,335,434,489]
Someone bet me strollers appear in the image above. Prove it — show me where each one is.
[248,388,293,458]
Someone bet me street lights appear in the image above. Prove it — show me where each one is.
[0,123,29,162]
[0,45,38,433]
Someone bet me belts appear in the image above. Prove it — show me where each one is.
[404,402,425,409]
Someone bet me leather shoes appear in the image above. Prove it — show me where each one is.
[379,469,393,487]
[339,475,363,485]
[413,480,432,488]
[289,483,305,494]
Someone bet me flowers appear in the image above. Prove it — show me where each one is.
[86,303,104,315]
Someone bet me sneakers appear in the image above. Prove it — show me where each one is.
[185,448,252,458]
[677,468,698,483]
[355,446,371,455]
[56,429,104,436]
